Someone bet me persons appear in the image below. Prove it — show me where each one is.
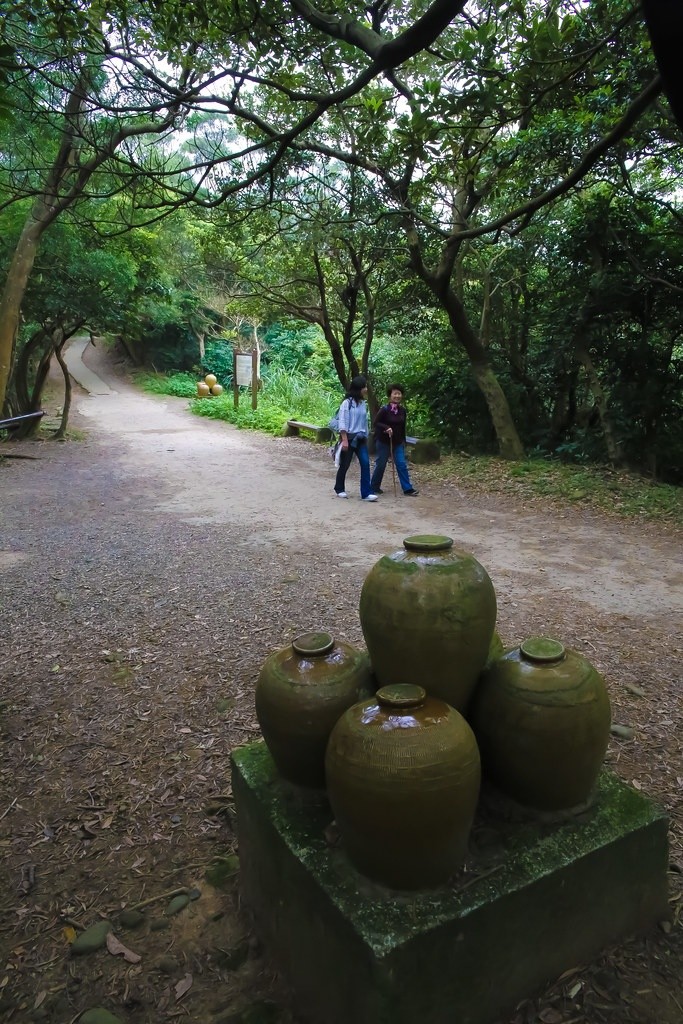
[367,383,421,495]
[332,376,378,502]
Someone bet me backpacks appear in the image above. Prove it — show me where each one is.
[327,407,340,434]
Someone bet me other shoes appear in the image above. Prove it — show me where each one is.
[404,489,419,496]
[374,489,382,494]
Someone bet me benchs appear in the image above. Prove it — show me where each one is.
[368,430,440,465]
[281,419,336,445]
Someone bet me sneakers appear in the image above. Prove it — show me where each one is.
[363,494,378,502]
[335,491,348,499]
